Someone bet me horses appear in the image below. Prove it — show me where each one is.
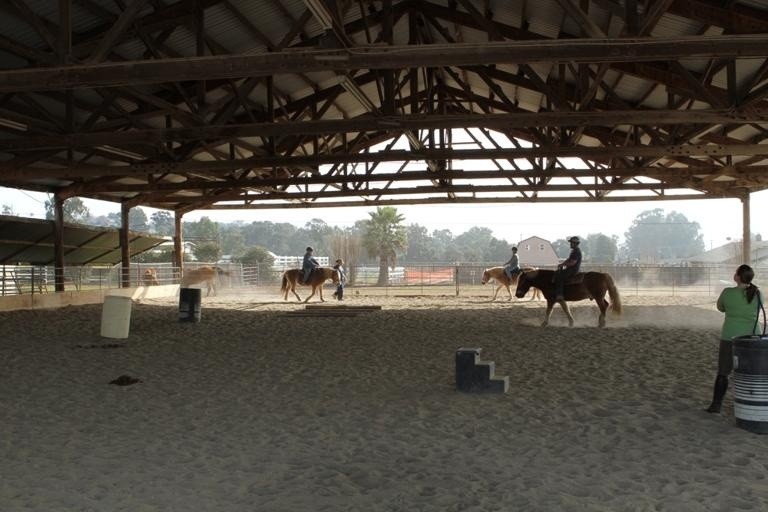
[515,269,621,328]
[279,267,342,304]
[179,266,233,298]
[481,265,541,302]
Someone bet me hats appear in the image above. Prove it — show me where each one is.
[568,236,581,243]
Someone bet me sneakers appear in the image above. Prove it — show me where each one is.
[702,407,720,414]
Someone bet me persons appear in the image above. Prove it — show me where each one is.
[503,247,520,285]
[704,264,765,413]
[331,259,346,300]
[550,237,582,300]
[301,247,319,284]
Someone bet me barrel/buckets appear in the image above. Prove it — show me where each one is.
[178,288,201,323]
[100,295,132,339]
[732,335,767,435]
